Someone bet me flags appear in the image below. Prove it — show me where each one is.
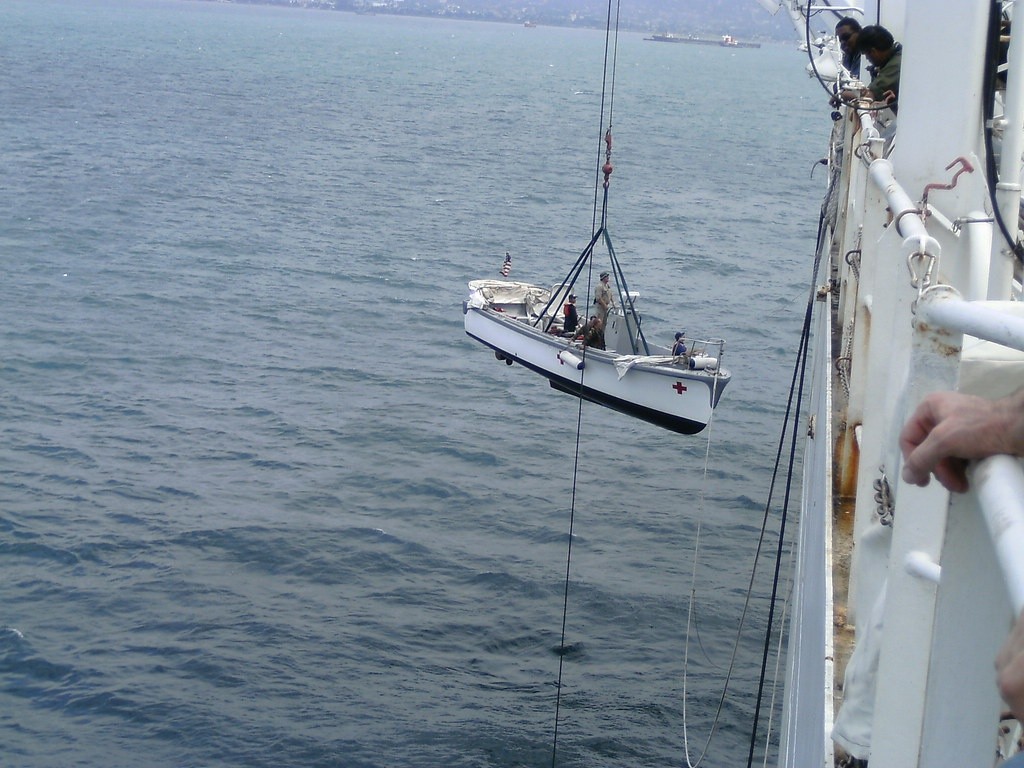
[499,252,511,277]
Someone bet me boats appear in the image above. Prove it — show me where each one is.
[643,30,762,49]
[460,249,733,436]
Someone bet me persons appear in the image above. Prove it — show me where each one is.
[672,332,687,355]
[900,382,1024,729]
[833,17,862,93]
[829,25,902,115]
[563,294,579,332]
[582,319,605,350]
[594,272,616,325]
[549,325,565,337]
[568,315,596,344]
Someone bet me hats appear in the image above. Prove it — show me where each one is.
[599,271,609,281]
[675,331,684,342]
[592,318,602,328]
[568,294,578,298]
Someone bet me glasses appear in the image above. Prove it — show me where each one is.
[838,31,856,42]
